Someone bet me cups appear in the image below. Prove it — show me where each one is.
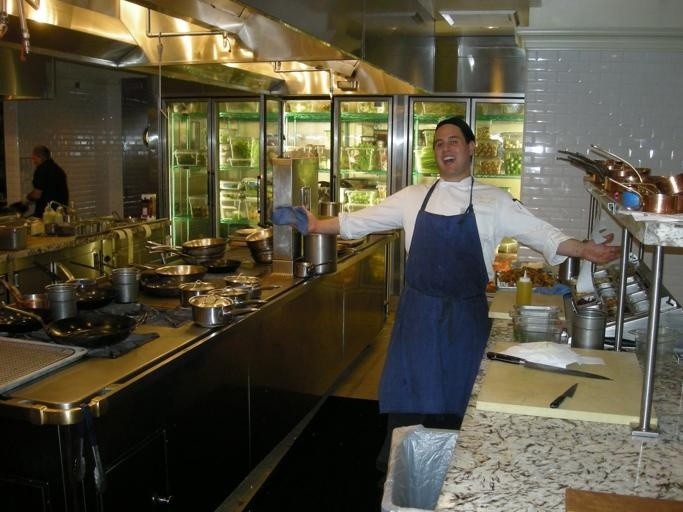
[572,309,606,349]
[628,324,677,377]
[112,266,140,304]
[43,283,79,321]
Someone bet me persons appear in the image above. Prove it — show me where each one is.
[25,145,70,217]
[287,117,621,491]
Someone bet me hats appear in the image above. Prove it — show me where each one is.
[434,116,476,145]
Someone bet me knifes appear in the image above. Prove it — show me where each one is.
[550,384,578,408]
[486,351,615,381]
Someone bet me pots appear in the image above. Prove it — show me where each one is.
[303,235,338,276]
[294,260,329,278]
[0,225,27,251]
[67,258,210,297]
[553,142,683,215]
[176,274,280,328]
[145,237,232,266]
[0,307,49,334]
[3,305,139,349]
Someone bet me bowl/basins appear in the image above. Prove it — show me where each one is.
[245,229,272,264]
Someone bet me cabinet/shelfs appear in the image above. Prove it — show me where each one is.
[155,92,525,290]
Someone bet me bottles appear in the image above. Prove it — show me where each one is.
[515,269,532,307]
[558,256,579,285]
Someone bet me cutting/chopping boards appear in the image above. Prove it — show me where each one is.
[487,289,567,322]
[476,341,657,427]
